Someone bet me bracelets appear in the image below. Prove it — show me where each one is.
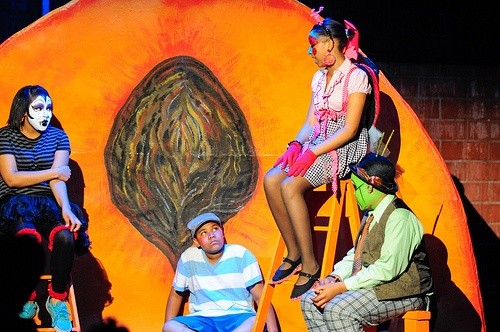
[325,275,340,284]
[286,140,303,148]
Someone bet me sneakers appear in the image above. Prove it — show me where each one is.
[18,302,38,319]
[46,295,73,332]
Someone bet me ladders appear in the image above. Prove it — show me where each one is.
[251,177,378,332]
[31,239,82,332]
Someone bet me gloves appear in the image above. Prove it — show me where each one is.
[274,140,303,169]
[287,152,314,178]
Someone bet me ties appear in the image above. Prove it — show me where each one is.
[352,215,374,276]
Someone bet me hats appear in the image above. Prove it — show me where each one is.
[349,152,398,194]
[187,212,221,238]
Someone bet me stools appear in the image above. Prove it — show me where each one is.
[397,310,431,332]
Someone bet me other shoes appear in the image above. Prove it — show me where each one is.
[290,265,322,299]
[272,255,302,285]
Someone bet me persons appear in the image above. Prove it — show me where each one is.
[300,153,432,332]
[0,85,89,332]
[263,6,381,297]
[162,213,279,332]
